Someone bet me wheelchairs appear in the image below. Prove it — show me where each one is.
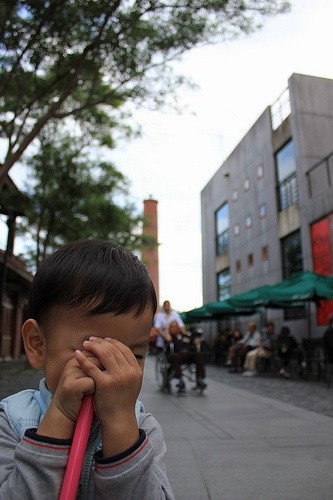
[154,334,208,395]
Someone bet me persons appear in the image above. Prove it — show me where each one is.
[0,238,175,500]
[154,300,186,394]
[320,315,333,389]
[222,320,301,378]
[166,320,206,390]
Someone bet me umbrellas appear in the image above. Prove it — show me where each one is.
[152,285,272,331]
[257,270,333,346]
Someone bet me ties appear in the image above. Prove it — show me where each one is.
[241,334,252,348]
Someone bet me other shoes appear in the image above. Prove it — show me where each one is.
[242,370,257,377]
[197,382,207,389]
[176,381,185,388]
[280,369,289,378]
